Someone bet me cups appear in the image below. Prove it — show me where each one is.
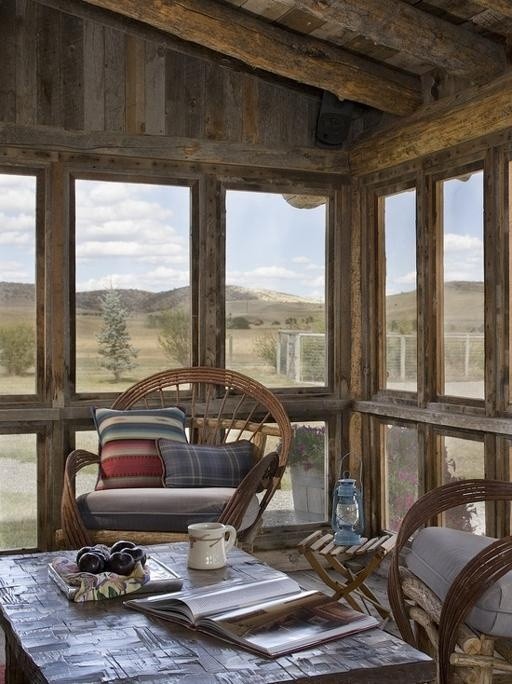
[188,522,237,570]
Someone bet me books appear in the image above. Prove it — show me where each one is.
[122,573,380,660]
[48,555,184,602]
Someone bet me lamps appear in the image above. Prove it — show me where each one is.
[331,452,365,545]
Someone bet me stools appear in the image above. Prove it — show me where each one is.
[296,529,398,632]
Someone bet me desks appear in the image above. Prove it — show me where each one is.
[0,541,438,684]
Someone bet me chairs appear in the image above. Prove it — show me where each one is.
[386,476,512,684]
[56,365,293,557]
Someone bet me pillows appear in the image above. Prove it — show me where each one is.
[89,404,258,491]
